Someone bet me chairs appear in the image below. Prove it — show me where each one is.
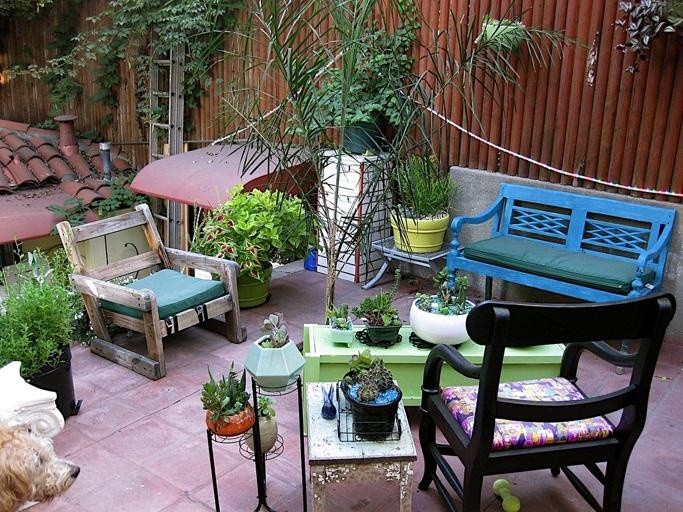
[419,292,676,512]
[56,203,247,380]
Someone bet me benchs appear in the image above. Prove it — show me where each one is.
[446,181,676,374]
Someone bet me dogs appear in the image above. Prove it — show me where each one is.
[0,422,80,512]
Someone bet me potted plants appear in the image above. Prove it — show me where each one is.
[285,0,420,155]
[213,182,323,309]
[0,230,84,419]
[391,154,457,253]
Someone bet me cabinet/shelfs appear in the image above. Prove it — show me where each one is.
[317,149,393,283]
[305,380,418,512]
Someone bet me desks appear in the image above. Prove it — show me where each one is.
[302,323,566,436]
